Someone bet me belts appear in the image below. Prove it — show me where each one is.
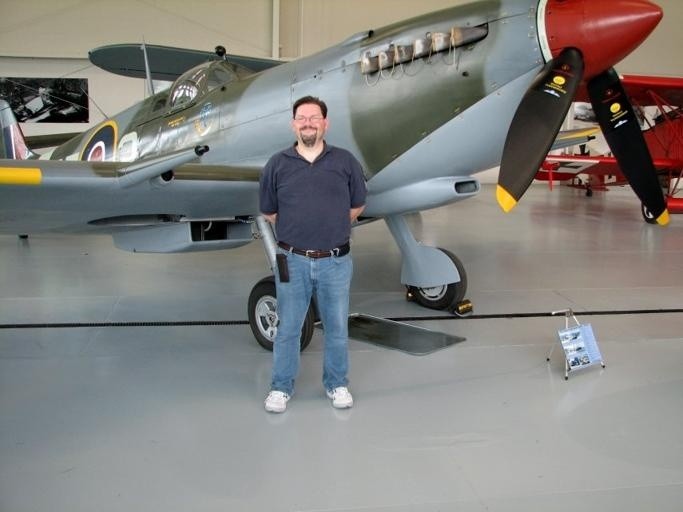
[277,240,350,258]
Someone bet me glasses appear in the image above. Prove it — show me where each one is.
[294,115,323,122]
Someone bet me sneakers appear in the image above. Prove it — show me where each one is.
[264,390,290,412]
[326,386,352,408]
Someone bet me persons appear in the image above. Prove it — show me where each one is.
[256,96,367,413]
